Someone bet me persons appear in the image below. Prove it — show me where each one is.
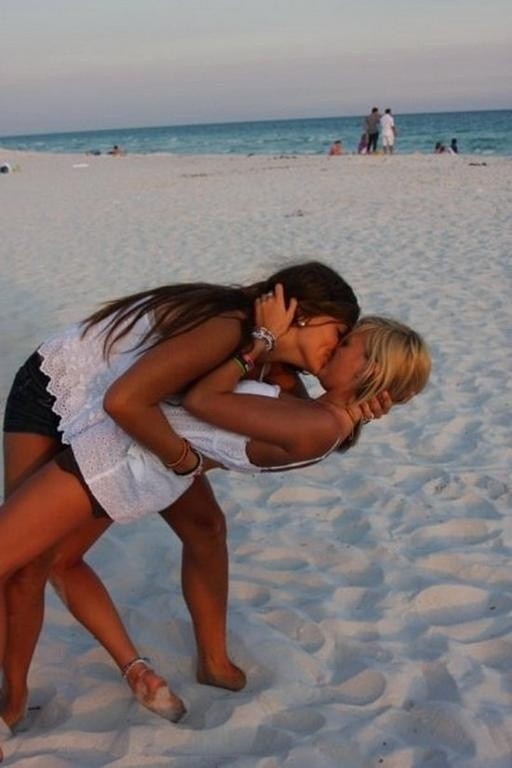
[84,144,125,156]
[328,106,460,157]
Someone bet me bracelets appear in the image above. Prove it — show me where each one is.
[233,353,254,377]
[253,327,275,351]
[166,438,189,468]
[175,450,203,479]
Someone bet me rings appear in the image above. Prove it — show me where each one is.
[267,292,272,297]
